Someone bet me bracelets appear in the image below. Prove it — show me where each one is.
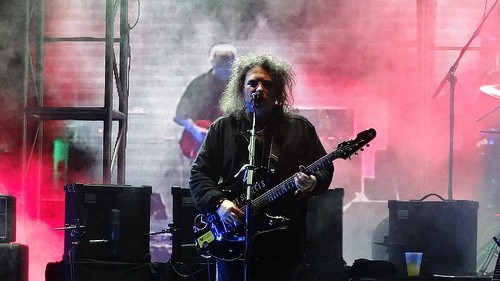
[215,198,226,208]
[307,182,314,190]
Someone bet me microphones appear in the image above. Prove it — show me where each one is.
[110,209,120,261]
[251,91,264,109]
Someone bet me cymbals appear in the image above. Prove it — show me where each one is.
[479,84,500,100]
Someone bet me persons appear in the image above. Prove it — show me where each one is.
[175,45,236,143]
[188,53,334,281]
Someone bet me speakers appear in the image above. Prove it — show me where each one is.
[304,188,344,264]
[170,185,213,263]
[0,243,29,281]
[386,198,479,276]
[63,183,152,261]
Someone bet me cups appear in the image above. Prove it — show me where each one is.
[404,253,423,276]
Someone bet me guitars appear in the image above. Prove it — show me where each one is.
[179,120,212,160]
[193,127,378,263]
